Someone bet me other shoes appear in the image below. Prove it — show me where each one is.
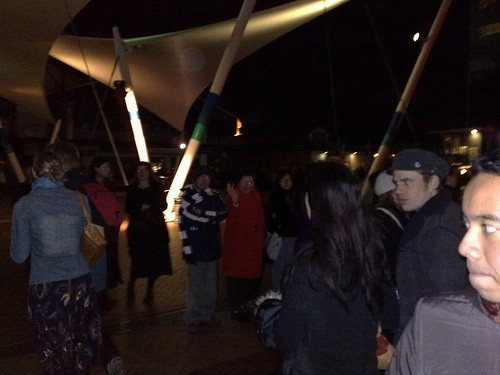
[231,307,256,322]
[127,281,137,303]
[199,318,219,327]
[144,294,155,307]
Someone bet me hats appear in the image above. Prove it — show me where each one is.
[393,149,450,180]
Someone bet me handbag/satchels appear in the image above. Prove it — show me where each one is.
[76,191,109,266]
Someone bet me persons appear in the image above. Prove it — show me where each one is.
[267,170,304,292]
[221,157,266,320]
[178,165,228,333]
[384,156,500,375]
[124,162,173,311]
[273,162,393,375]
[389,150,469,347]
[10,151,103,375]
[46,141,124,375]
